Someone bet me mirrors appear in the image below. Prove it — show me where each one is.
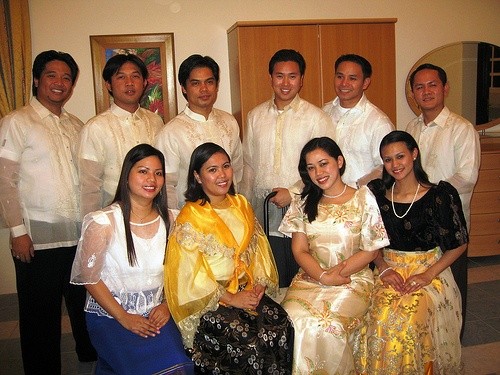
[405,41,500,136]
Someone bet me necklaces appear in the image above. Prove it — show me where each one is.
[322,184,346,199]
[131,207,152,224]
[392,179,422,218]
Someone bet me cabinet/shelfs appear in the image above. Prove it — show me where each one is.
[466,133,500,257]
[226,17,398,144]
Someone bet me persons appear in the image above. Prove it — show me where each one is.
[241,49,336,288]
[157,54,242,223]
[277,137,390,375]
[366,131,469,375]
[402,63,481,345]
[77,55,163,221]
[68,144,196,375]
[166,145,294,375]
[320,54,397,192]
[0,51,98,375]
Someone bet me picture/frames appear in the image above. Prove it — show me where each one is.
[89,33,178,125]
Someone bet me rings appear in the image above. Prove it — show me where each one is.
[413,281,416,285]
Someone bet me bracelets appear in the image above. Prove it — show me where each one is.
[380,267,393,277]
[318,272,327,285]
[357,182,361,189]
